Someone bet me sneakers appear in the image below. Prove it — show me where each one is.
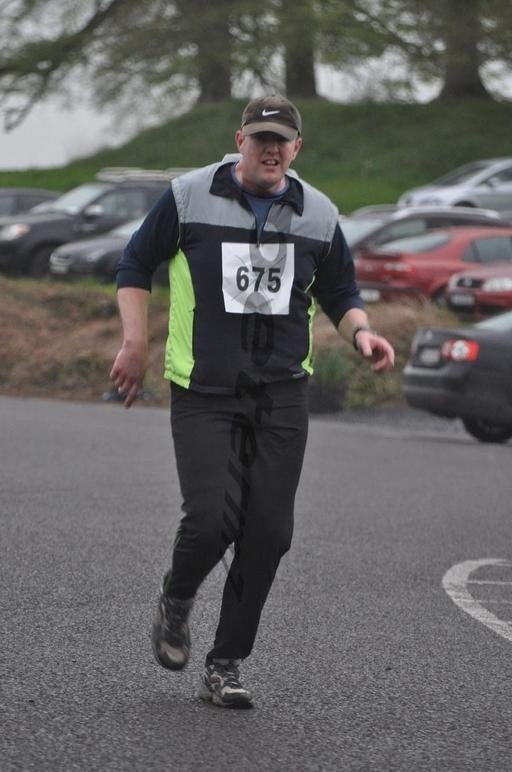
[197,659,251,708]
[150,570,195,671]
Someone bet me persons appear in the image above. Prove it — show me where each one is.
[109,95,396,709]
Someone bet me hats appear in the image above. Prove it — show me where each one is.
[242,93,302,140]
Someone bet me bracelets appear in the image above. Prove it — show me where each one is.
[353,326,371,350]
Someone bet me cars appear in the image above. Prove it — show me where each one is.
[400,307,511,443]
